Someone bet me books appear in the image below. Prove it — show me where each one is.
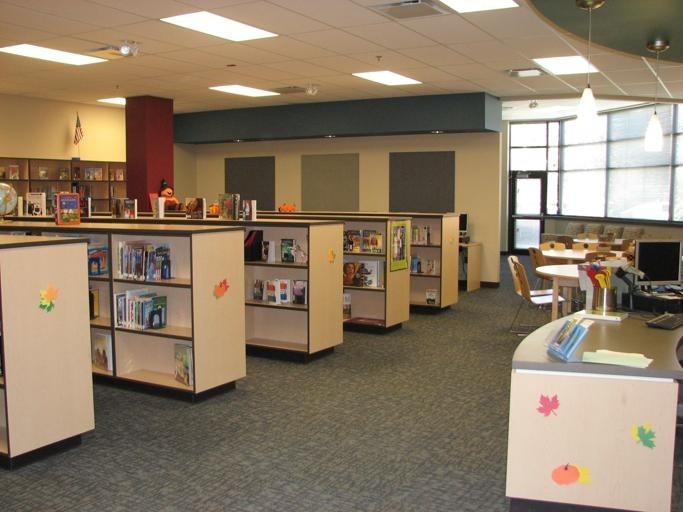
[340,221,440,329]
[123,198,138,220]
[24,191,47,215]
[53,191,82,226]
[86,241,194,390]
[14,196,22,217]
[251,238,308,308]
[154,196,165,220]
[85,197,93,218]
[241,199,258,222]
[1,164,124,198]
[216,192,240,221]
[184,197,206,219]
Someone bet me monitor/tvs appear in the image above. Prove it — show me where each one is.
[459,213,468,238]
[633,239,682,296]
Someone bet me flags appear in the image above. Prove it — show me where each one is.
[70,115,85,145]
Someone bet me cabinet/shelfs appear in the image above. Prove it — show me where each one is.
[3,213,345,364]
[409,213,461,314]
[124,211,413,336]
[0,156,127,213]
[3,219,249,402]
[0,236,97,470]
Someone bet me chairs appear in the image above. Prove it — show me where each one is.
[507,220,651,335]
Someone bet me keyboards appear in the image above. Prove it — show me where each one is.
[646,312,683,330]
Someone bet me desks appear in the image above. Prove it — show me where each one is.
[459,241,481,293]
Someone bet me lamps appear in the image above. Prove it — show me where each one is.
[576,1,606,105]
[642,39,670,154]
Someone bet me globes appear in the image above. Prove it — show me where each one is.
[0,182,18,225]
